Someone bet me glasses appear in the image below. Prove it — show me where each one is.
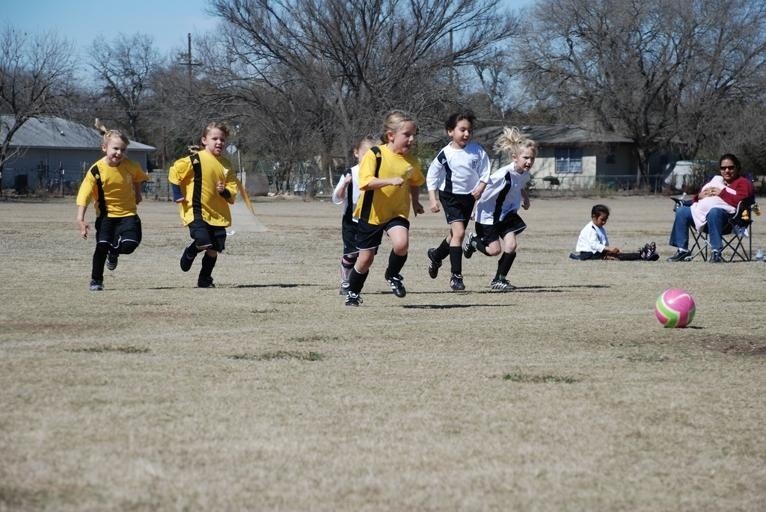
[718,165,738,171]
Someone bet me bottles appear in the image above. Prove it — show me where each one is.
[756,246,763,267]
[672,192,687,215]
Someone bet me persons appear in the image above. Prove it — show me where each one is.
[331,134,378,296]
[667,153,754,263]
[464,125,538,290]
[427,109,491,290]
[76,129,150,292]
[168,121,238,288]
[345,109,426,307]
[575,205,656,261]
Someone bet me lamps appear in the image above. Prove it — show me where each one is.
[674,207,752,262]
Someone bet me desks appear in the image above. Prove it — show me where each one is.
[670,194,700,207]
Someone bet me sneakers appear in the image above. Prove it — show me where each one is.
[449,273,465,291]
[639,242,651,261]
[339,279,352,296]
[427,247,442,279]
[105,244,118,271]
[196,277,215,289]
[343,290,361,308]
[464,232,479,259]
[666,248,692,263]
[491,274,517,292]
[648,241,657,260]
[707,250,722,263]
[90,279,104,291]
[180,247,195,272]
[384,267,407,298]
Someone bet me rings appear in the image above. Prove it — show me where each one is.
[710,190,711,193]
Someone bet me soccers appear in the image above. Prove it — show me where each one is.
[656,287,695,328]
